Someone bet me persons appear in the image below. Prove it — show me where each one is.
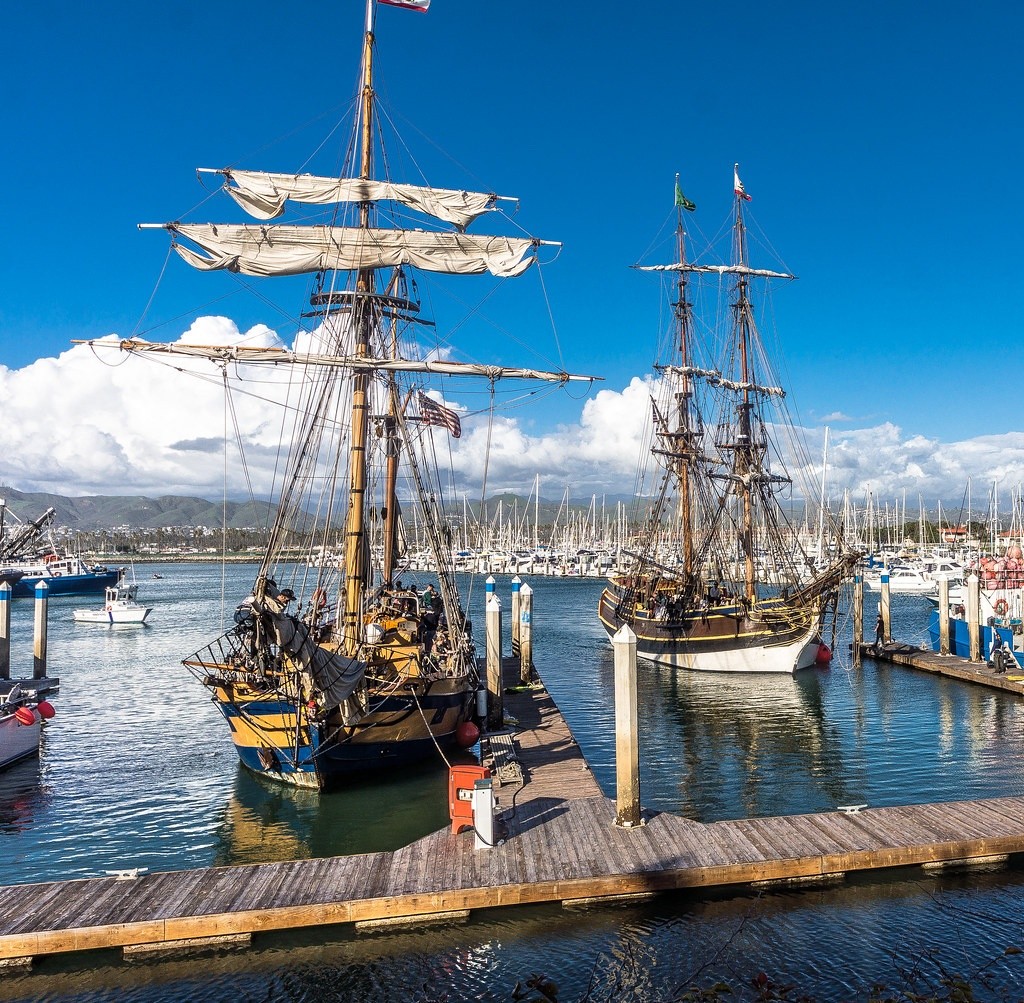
[870,614,884,646]
[728,585,738,598]
[278,583,435,608]
[710,582,722,599]
[990,634,1005,675]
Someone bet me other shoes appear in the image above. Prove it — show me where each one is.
[993,672,999,674]
[998,671,1004,674]
[879,644,884,647]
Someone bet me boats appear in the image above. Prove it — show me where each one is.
[72,572,153,623]
[0,497,127,599]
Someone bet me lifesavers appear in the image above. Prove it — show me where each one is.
[992,597,1009,615]
[313,587,327,610]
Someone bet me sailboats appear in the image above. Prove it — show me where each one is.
[596,162,869,676]
[69,0,608,795]
[301,471,991,594]
[926,475,1024,669]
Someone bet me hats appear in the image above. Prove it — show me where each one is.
[281,589,296,601]
[411,585,417,588]
[429,584,435,588]
